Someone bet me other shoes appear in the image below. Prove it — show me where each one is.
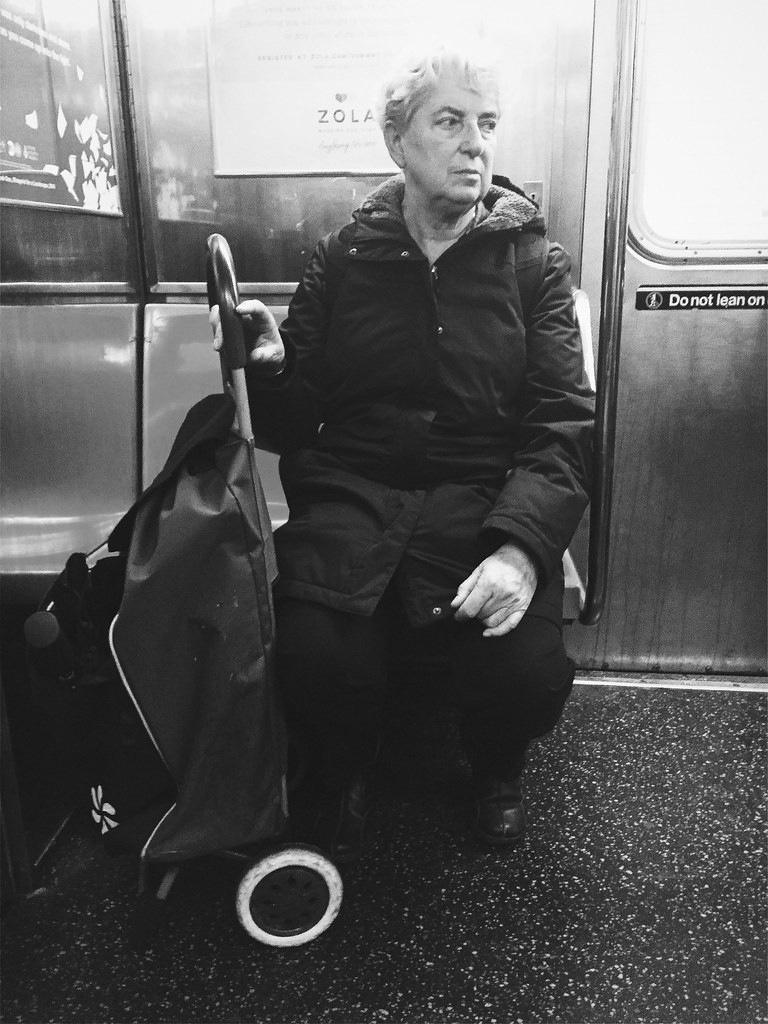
[498,782,526,845]
[309,783,384,861]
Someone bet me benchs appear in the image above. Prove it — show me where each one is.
[0,289,613,625]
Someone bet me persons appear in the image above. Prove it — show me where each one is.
[205,57,598,844]
